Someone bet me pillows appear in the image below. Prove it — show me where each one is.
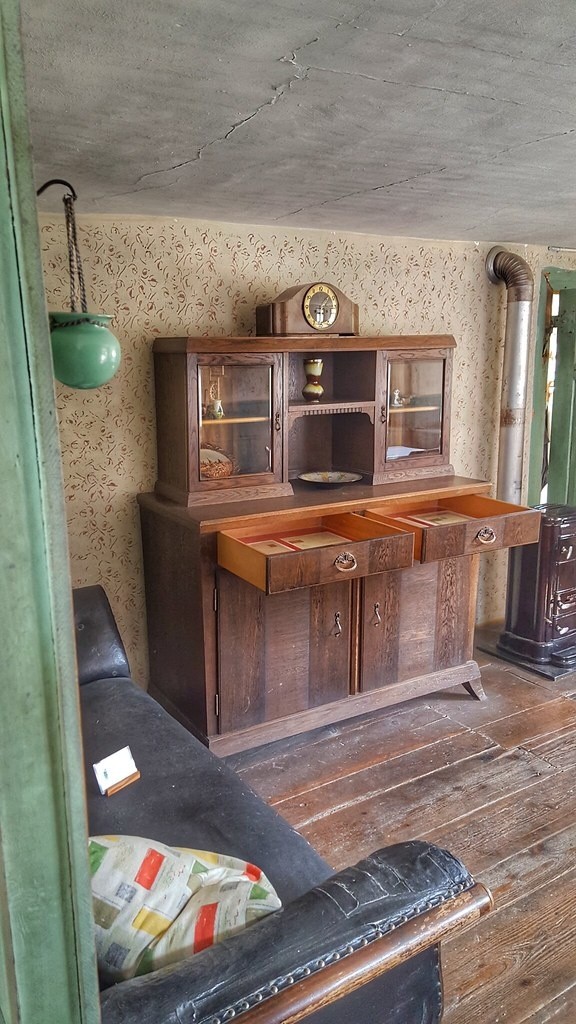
[83,832,283,983]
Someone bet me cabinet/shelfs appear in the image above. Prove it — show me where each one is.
[136,336,544,758]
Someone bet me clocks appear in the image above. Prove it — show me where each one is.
[303,283,341,330]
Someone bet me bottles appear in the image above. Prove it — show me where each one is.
[213,399,224,420]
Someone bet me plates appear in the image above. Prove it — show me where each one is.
[297,469,363,490]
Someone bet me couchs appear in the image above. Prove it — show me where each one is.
[70,584,494,1024]
